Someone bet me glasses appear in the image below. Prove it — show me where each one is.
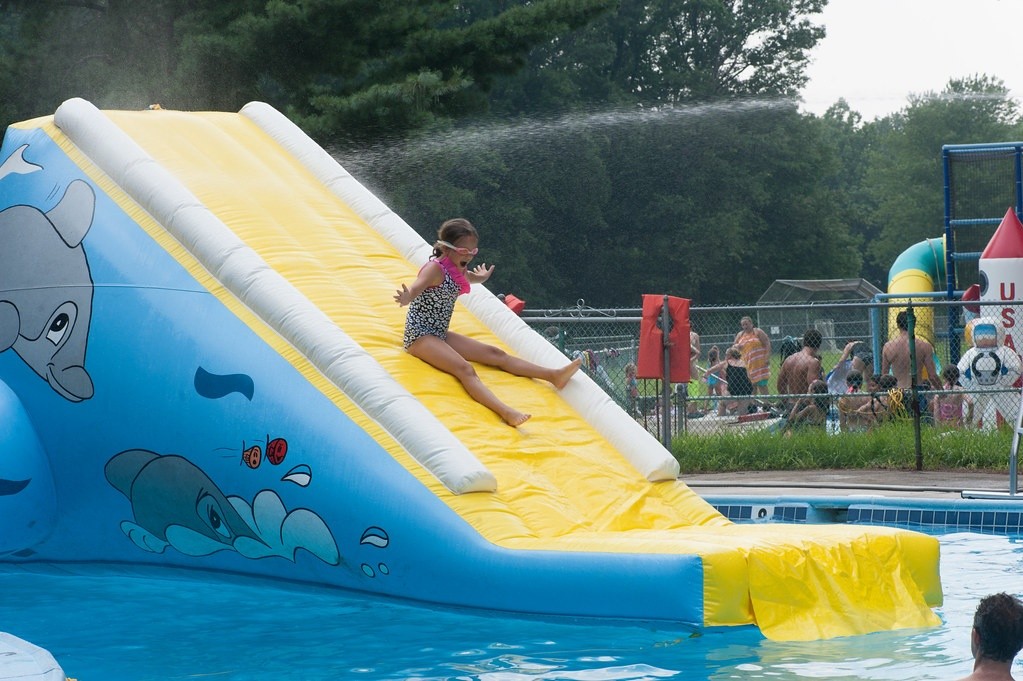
[454,246,479,255]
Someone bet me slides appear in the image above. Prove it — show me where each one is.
[886,236,945,353]
[1,97,945,642]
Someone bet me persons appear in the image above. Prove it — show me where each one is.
[954,590,1023,680]
[393,216,582,426]
[622,311,975,438]
